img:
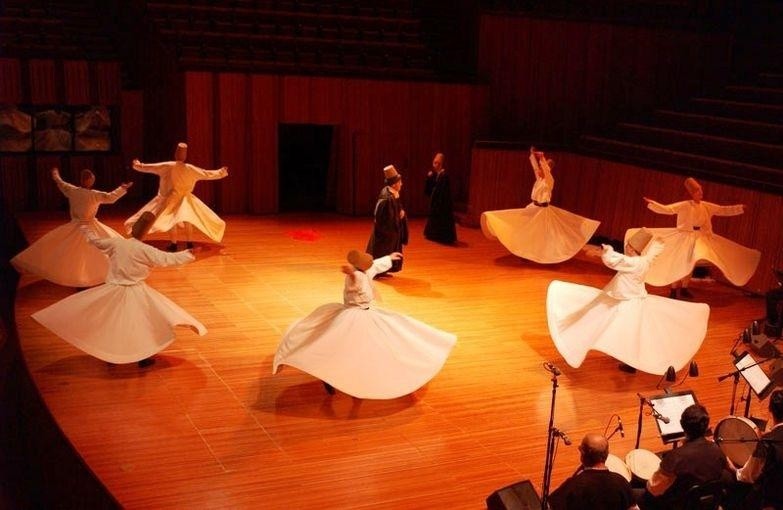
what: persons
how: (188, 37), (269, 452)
(425, 153), (456, 244)
(271, 251), (456, 399)
(124, 143), (229, 251)
(29, 211), (207, 368)
(367, 164), (409, 280)
(622, 177), (762, 300)
(632, 403), (726, 509)
(545, 431), (632, 509)
(547, 227), (712, 375)
(727, 387), (783, 510)
(480, 145), (601, 265)
(10, 167), (134, 292)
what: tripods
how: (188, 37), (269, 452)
(741, 388), (752, 418)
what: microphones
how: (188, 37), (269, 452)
(645, 409), (668, 424)
(543, 362), (561, 376)
(551, 428), (573, 446)
(617, 416), (624, 437)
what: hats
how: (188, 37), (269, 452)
(174, 142), (188, 160)
(132, 211), (156, 241)
(80, 169), (95, 188)
(628, 227), (653, 253)
(383, 165), (399, 179)
(539, 159), (555, 172)
(684, 177), (701, 195)
(347, 250), (374, 272)
